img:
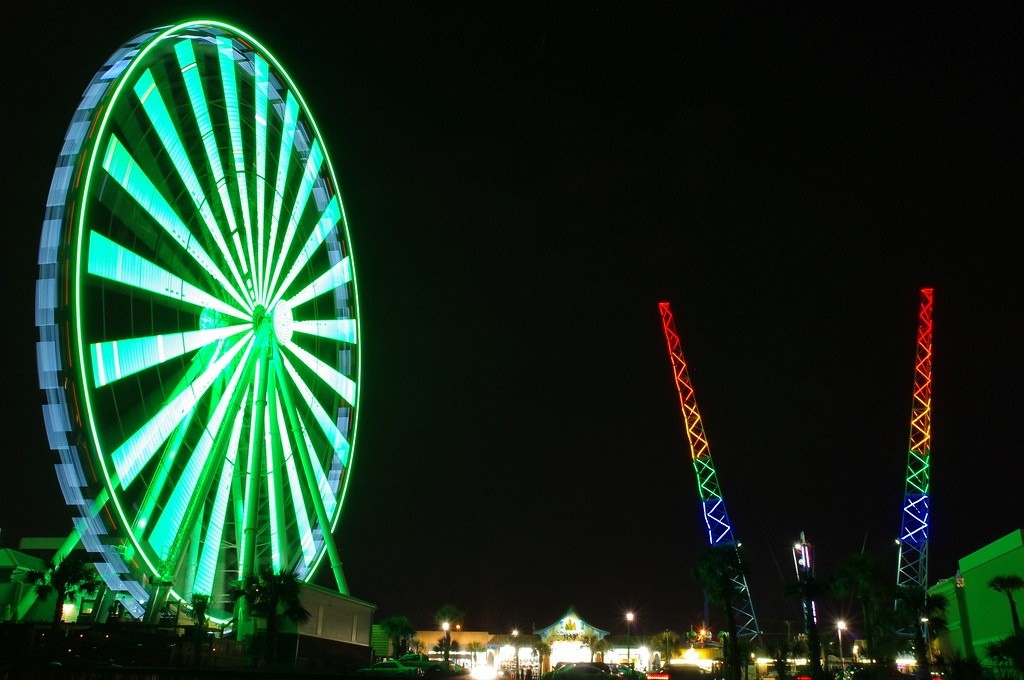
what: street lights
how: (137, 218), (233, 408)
(442, 622), (450, 662)
(511, 628), (520, 680)
(836, 618), (846, 668)
(626, 611), (633, 662)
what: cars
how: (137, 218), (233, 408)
(359, 654), (463, 680)
(542, 661), (715, 680)
(835, 662), (912, 680)
